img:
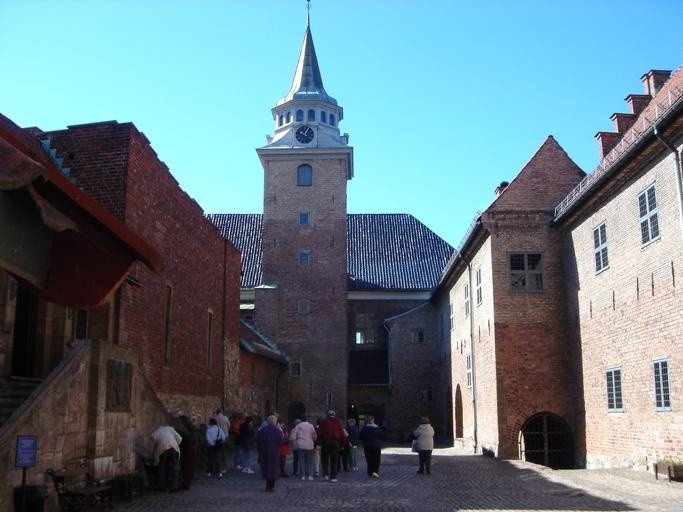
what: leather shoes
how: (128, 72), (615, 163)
(265, 487), (274, 492)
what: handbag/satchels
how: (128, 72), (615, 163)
(216, 439), (223, 450)
(412, 439), (416, 452)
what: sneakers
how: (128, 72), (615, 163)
(301, 476), (305, 480)
(368, 472), (379, 478)
(237, 464), (255, 474)
(324, 474), (338, 482)
(417, 470), (430, 474)
(309, 476), (313, 480)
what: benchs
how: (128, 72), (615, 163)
(47, 466), (114, 511)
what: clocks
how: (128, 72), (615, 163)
(289, 123), (319, 150)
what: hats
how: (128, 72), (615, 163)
(327, 410), (335, 415)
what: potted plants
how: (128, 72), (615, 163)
(653, 458), (683, 481)
(117, 471), (145, 501)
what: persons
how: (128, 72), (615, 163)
(150, 409), (434, 492)
